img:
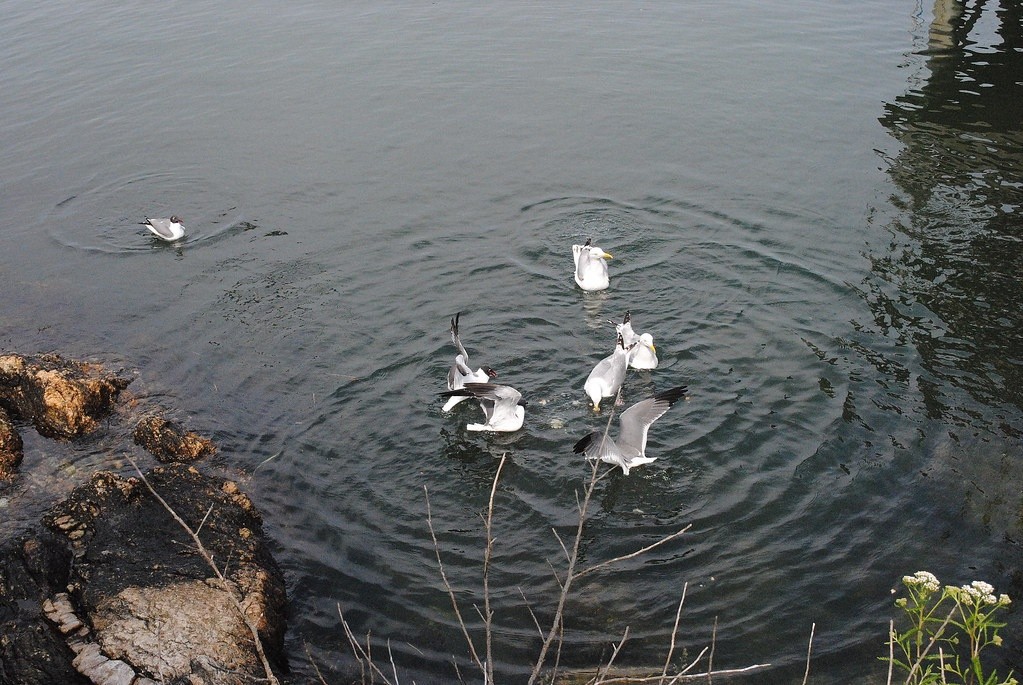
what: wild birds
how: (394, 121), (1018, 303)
(435, 382), (528, 437)
(583, 332), (638, 411)
(572, 238), (614, 292)
(436, 311), (496, 412)
(607, 310), (658, 369)
(138, 215), (186, 242)
(573, 385), (689, 476)
